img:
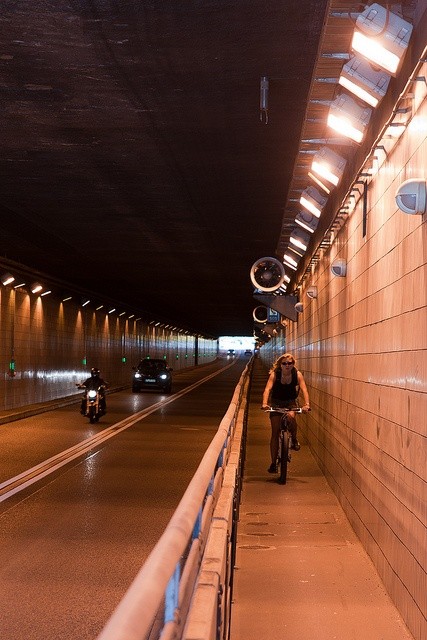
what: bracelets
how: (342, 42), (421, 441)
(304, 403), (310, 406)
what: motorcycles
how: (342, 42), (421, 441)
(76, 384), (105, 423)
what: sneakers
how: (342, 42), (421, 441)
(292, 439), (300, 451)
(268, 463), (277, 473)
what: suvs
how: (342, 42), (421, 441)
(132, 359), (172, 392)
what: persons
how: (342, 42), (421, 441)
(80, 367), (108, 415)
(261, 353), (311, 473)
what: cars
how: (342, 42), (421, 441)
(244, 349), (252, 356)
(227, 349), (235, 355)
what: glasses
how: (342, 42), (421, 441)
(281, 361), (293, 365)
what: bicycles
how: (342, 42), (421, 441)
(260, 407), (311, 483)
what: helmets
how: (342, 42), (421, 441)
(91, 368), (101, 377)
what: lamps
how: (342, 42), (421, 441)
(290, 228), (310, 252)
(350, 4), (413, 79)
(295, 211), (319, 234)
(328, 94), (372, 145)
(339, 56), (391, 109)
(311, 147), (347, 188)
(300, 186), (329, 218)
(283, 250), (301, 268)
